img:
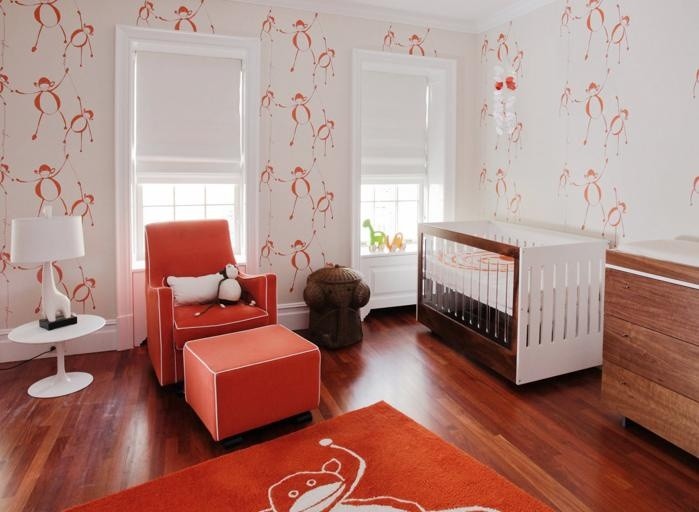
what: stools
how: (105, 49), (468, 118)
(182, 323), (321, 450)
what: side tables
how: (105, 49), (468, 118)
(7, 313), (106, 399)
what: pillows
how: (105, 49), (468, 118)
(163, 272), (224, 305)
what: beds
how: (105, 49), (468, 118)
(415, 219), (610, 387)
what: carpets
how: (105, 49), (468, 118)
(60, 399), (559, 512)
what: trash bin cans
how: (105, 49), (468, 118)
(303, 264), (369, 350)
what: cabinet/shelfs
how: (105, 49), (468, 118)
(600, 235), (698, 458)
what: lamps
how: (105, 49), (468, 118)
(8, 204), (85, 330)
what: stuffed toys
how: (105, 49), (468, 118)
(195, 263), (256, 317)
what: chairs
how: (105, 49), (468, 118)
(144, 219), (277, 387)
